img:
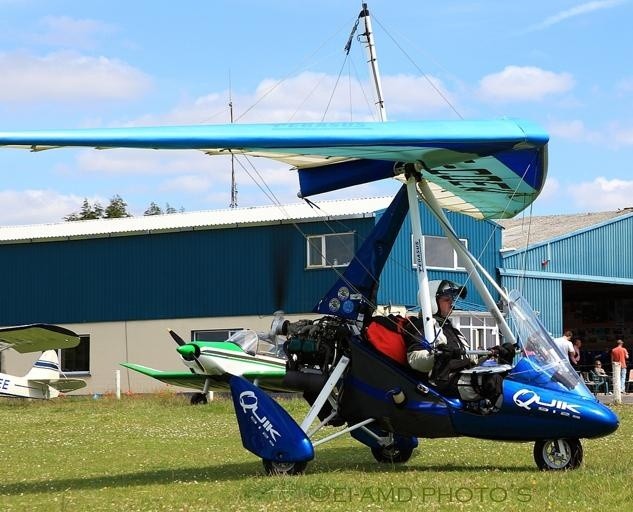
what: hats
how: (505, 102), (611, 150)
(617, 340), (624, 344)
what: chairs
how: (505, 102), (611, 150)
(576, 368), (633, 398)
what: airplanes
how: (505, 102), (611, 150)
(115, 324), (306, 405)
(0, 323), (89, 404)
(0, 3), (620, 477)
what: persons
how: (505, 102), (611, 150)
(609, 339), (629, 396)
(404, 277), (514, 418)
(593, 359), (608, 378)
(569, 338), (582, 371)
(592, 344), (612, 363)
(553, 329), (577, 366)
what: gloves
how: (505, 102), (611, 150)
(491, 345), (507, 359)
(446, 347), (465, 361)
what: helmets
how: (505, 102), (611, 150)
(417, 279), (467, 315)
